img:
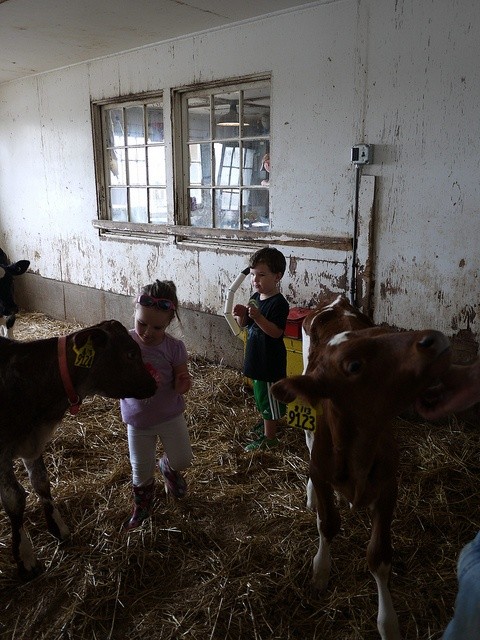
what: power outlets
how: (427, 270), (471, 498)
(351, 144), (373, 164)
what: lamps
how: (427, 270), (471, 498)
(216, 102), (248, 127)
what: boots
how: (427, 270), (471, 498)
(129, 478), (155, 528)
(159, 450), (187, 498)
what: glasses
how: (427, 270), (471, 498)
(140, 295), (176, 312)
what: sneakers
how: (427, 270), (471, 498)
(254, 424), (283, 437)
(243, 435), (278, 453)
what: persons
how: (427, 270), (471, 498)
(119, 280), (192, 531)
(233, 248), (289, 453)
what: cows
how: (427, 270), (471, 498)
(267, 293), (454, 640)
(0, 318), (158, 578)
(0, 247), (31, 340)
